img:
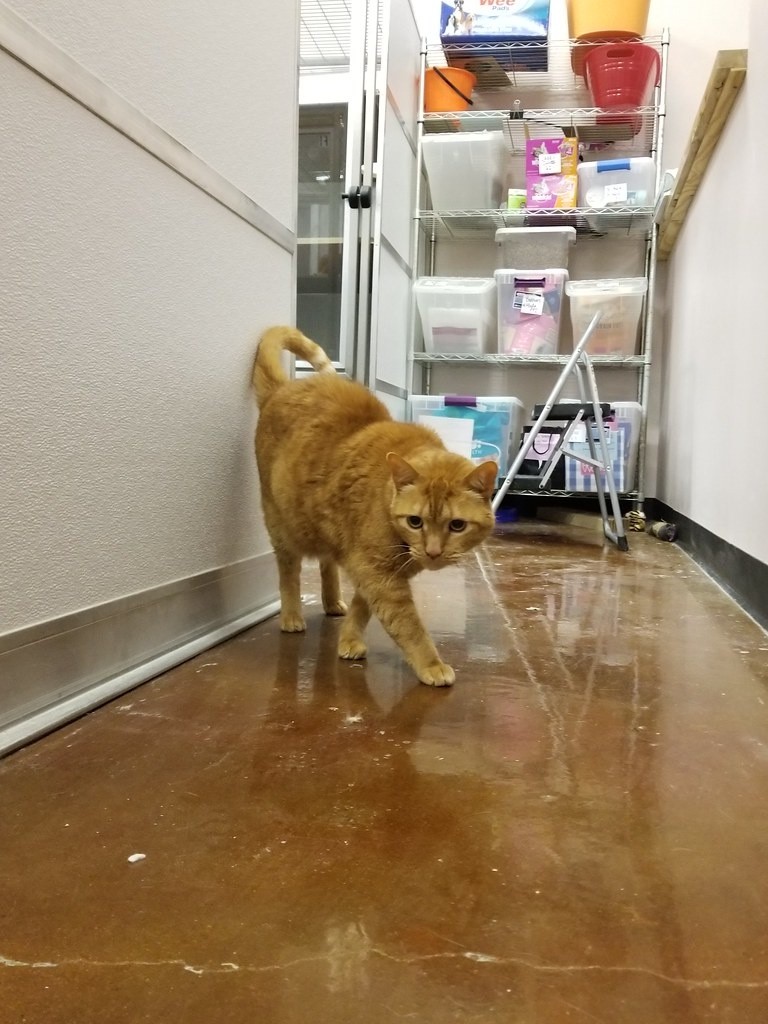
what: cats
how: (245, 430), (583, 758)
(252, 327), (497, 688)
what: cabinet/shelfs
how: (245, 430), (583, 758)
(405, 27), (669, 534)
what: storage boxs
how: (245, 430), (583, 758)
(492, 267), (570, 355)
(439, 0), (550, 72)
(526, 177), (578, 209)
(494, 226), (576, 272)
(418, 131), (509, 236)
(407, 394), (524, 476)
(566, 0), (650, 76)
(576, 155), (657, 226)
(563, 277), (649, 355)
(582, 43), (660, 124)
(559, 398), (642, 492)
(525, 137), (578, 176)
(411, 275), (497, 353)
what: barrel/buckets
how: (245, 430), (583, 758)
(565, 0), (650, 76)
(424, 66), (476, 112)
(581, 44), (661, 134)
